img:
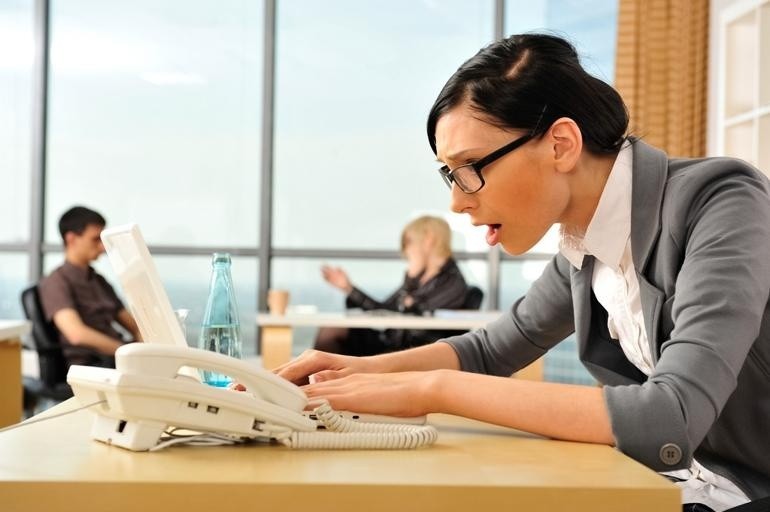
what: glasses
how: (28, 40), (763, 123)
(438, 129), (541, 194)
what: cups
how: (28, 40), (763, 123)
(265, 289), (289, 318)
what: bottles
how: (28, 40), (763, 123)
(198, 253), (241, 388)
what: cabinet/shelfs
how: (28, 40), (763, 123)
(0, 394), (683, 511)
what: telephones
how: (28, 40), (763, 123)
(67, 342), (316, 451)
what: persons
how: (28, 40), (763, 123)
(310, 214), (469, 357)
(37, 205), (145, 373)
(260, 32), (769, 512)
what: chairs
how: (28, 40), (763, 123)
(22, 286), (75, 400)
(412, 287), (482, 347)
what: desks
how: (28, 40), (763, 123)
(256, 311), (543, 380)
(1, 320), (32, 428)
